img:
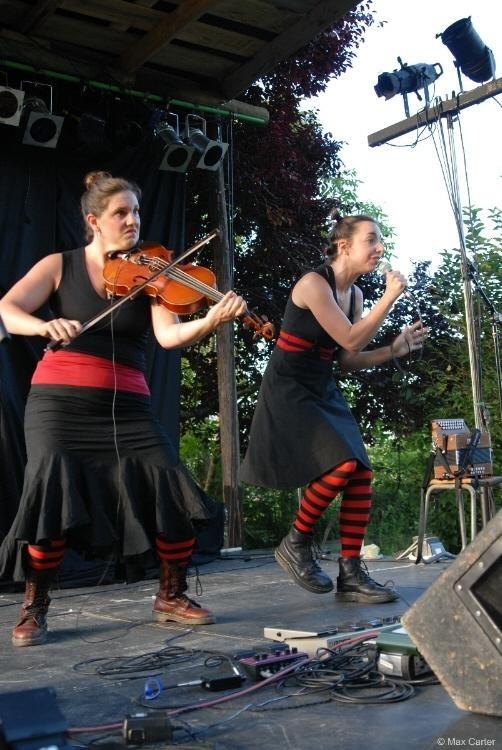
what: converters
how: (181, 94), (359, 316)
(201, 673), (242, 692)
(124, 712), (172, 745)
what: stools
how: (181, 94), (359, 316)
(414, 474), (502, 565)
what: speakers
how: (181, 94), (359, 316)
(0, 688), (69, 750)
(401, 508), (502, 716)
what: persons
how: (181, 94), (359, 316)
(237, 211), (435, 607)
(0, 170), (249, 647)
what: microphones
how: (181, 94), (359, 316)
(379, 262), (416, 303)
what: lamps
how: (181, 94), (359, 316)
(368, 16), (502, 147)
(0, 58), (266, 173)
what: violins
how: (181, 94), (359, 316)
(104, 241), (277, 338)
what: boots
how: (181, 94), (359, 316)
(10, 567), (53, 647)
(150, 558), (219, 626)
(273, 523), (334, 594)
(334, 556), (402, 604)
